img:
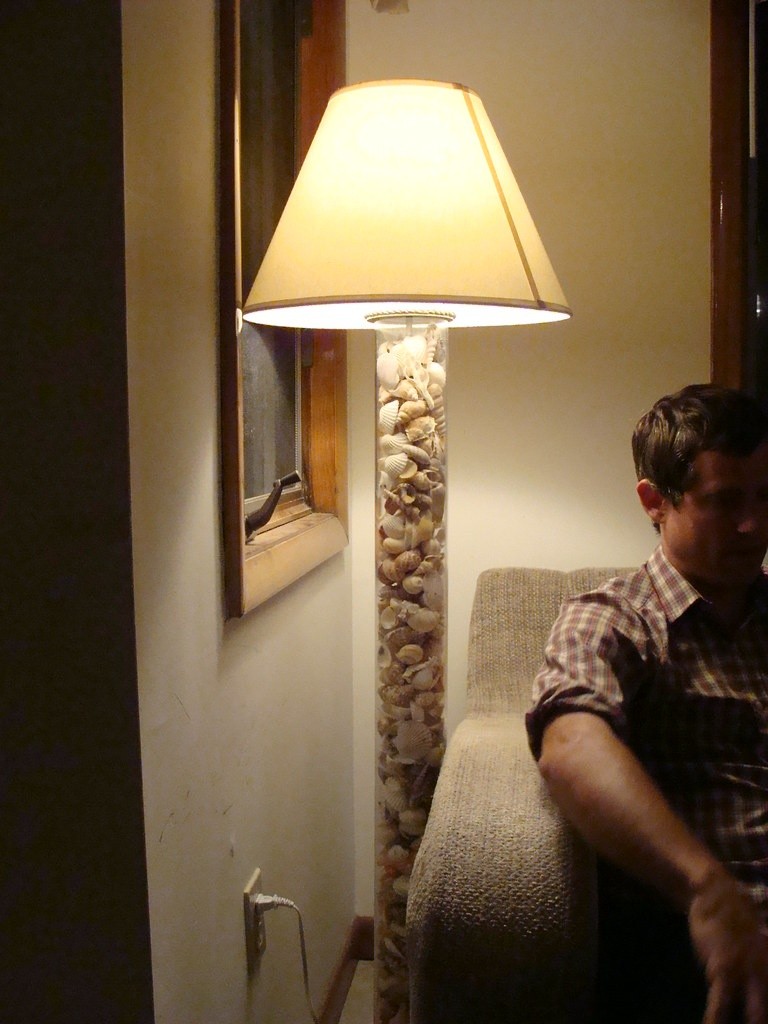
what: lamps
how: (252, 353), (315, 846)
(245, 80), (566, 1015)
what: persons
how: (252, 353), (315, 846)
(526, 383), (768, 1024)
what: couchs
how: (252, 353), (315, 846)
(406, 568), (654, 1021)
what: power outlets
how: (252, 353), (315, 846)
(243, 867), (275, 970)
